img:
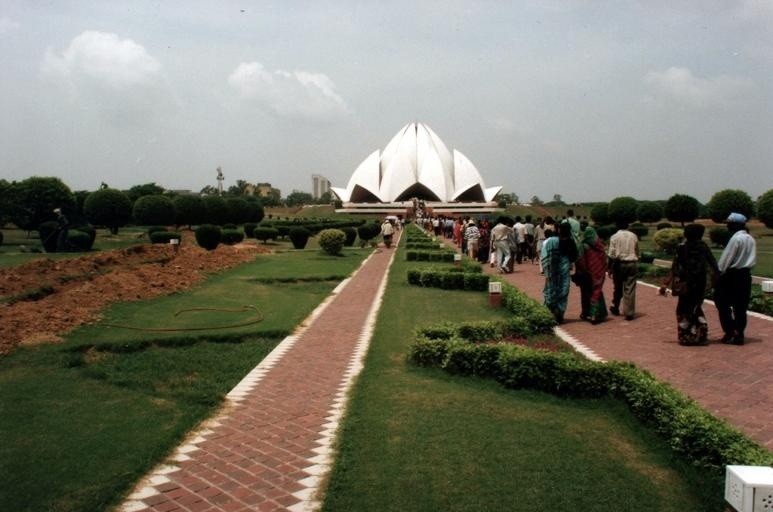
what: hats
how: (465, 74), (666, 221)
(726, 213), (746, 223)
(468, 219), (475, 225)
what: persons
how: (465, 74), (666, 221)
(540, 226), (578, 323)
(608, 216), (643, 321)
(715, 211), (757, 346)
(379, 195), (600, 275)
(570, 225), (608, 325)
(654, 222), (720, 346)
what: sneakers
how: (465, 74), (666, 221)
(610, 306), (619, 315)
(720, 331), (744, 345)
(591, 318), (597, 325)
(625, 315), (633, 320)
